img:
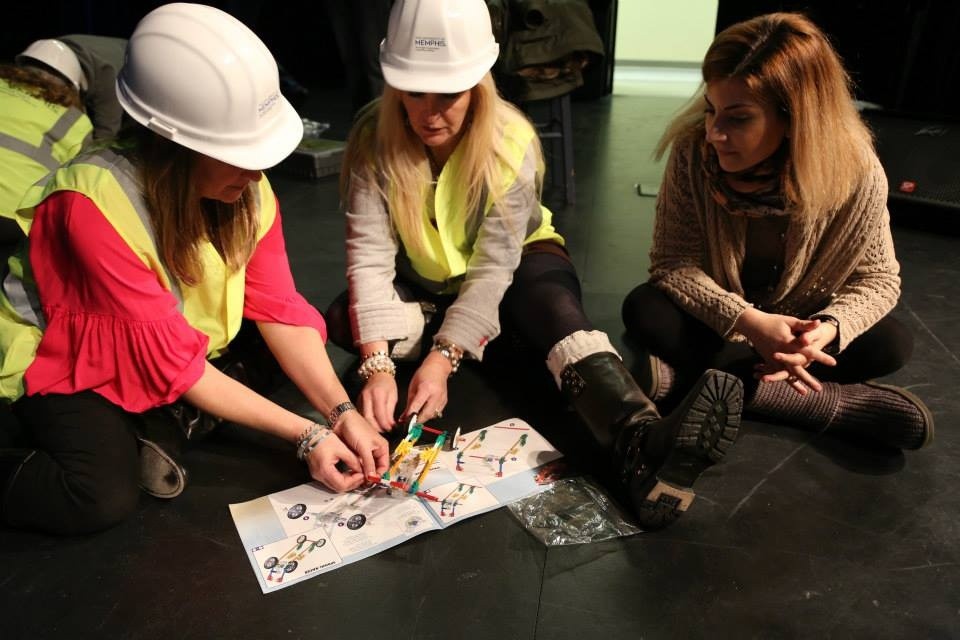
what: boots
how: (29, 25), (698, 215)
(138, 344), (253, 498)
(743, 374), (933, 451)
(390, 300), (532, 364)
(645, 354), (696, 405)
(543, 327), (743, 529)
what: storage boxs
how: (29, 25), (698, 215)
(884, 134), (959, 242)
(268, 135), (347, 180)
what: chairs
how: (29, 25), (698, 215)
(486, 1), (585, 208)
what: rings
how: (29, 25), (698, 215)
(435, 408), (443, 419)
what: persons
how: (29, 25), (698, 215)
(0, 37), (96, 292)
(52, 32), (131, 153)
(0, 6), (389, 539)
(326, 0), (746, 535)
(620, 16), (935, 453)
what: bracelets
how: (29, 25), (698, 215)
(296, 422), (333, 470)
(324, 402), (358, 428)
(358, 351), (397, 382)
(427, 336), (464, 381)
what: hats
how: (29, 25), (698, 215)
(15, 38), (81, 97)
(380, 0), (501, 94)
(116, 2), (304, 169)
